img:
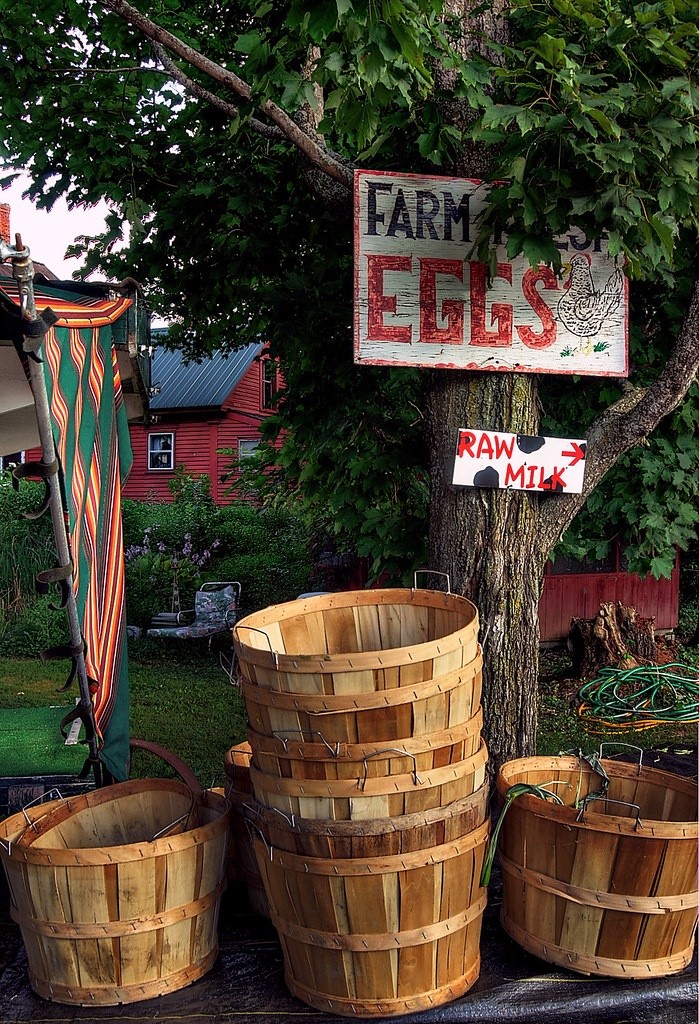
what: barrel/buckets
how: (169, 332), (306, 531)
(241, 772), (487, 860)
(231, 589), (480, 694)
(244, 703), (482, 780)
(249, 736), (489, 821)
(16, 778), (197, 848)
(0, 778), (232, 1008)
(247, 818), (490, 1017)
(235, 644), (484, 743)
(495, 758), (698, 979)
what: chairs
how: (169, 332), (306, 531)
(147, 581), (256, 655)
